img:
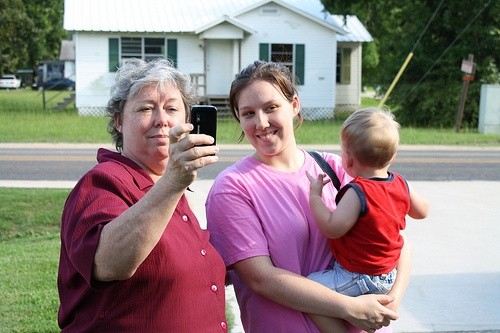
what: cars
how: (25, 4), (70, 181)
(0, 75), (21, 90)
(31, 78), (76, 91)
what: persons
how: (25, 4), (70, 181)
(303, 107), (429, 333)
(204, 59), (411, 333)
(57, 57), (228, 333)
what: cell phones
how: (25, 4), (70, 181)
(189, 105), (217, 157)
